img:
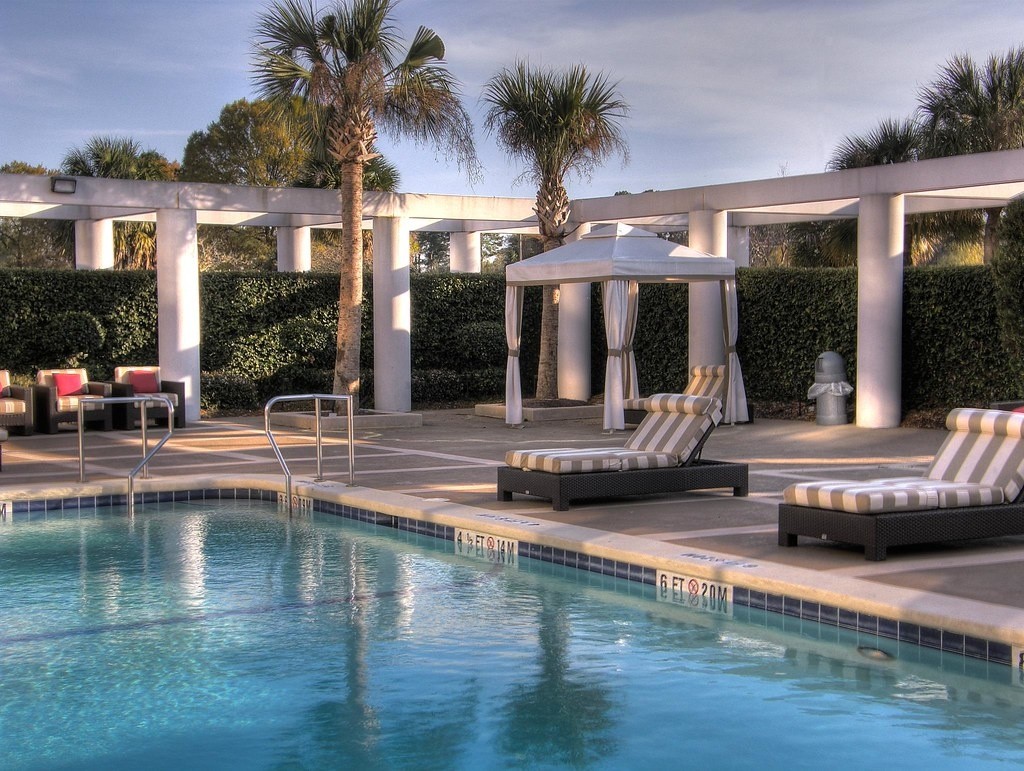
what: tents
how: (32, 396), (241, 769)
(505, 221), (750, 427)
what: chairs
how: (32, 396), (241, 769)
(0, 370), (33, 437)
(35, 367), (113, 434)
(497, 393), (749, 511)
(624, 364), (726, 428)
(777, 408), (1024, 562)
(104, 366), (186, 431)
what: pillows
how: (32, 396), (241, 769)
(52, 373), (82, 396)
(0, 381), (4, 399)
(128, 371), (159, 393)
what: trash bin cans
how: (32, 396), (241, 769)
(813, 351), (849, 424)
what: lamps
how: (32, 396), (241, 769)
(50, 176), (77, 194)
